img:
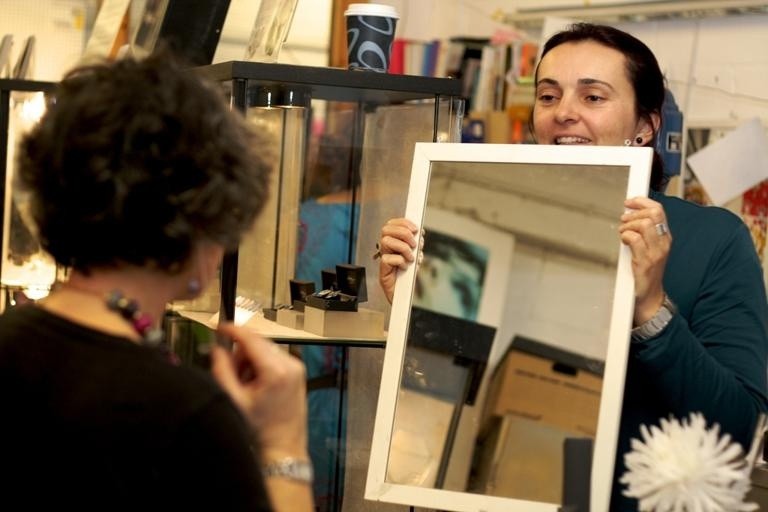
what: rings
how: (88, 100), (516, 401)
(369, 241), (384, 258)
(652, 222), (670, 238)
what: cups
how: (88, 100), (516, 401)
(343, 3), (399, 76)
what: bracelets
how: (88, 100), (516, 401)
(630, 292), (681, 345)
(244, 452), (320, 485)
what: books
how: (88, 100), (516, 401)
(386, 31), (541, 118)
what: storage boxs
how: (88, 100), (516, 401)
(481, 334), (606, 440)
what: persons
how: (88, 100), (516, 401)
(1, 55), (317, 511)
(377, 21), (768, 510)
(287, 134), (363, 512)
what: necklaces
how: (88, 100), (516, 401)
(41, 275), (184, 372)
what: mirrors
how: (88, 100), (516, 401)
(362, 139), (656, 512)
